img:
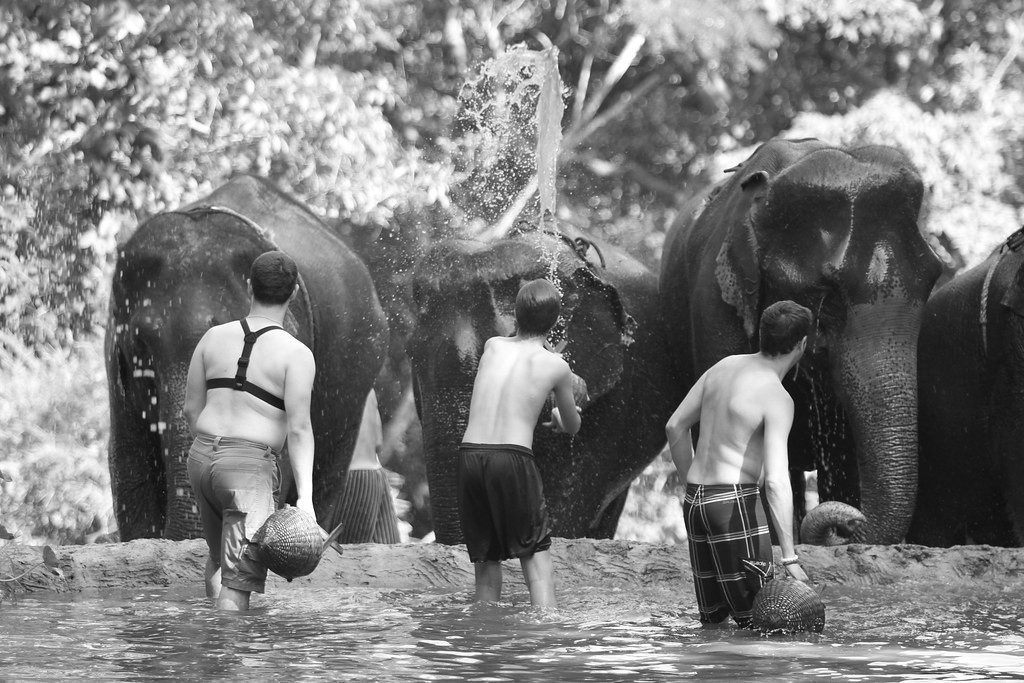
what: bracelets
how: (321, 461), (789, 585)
(781, 556), (798, 566)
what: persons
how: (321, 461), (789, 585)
(182, 251), (318, 611)
(456, 279), (582, 613)
(329, 386), (400, 544)
(666, 300), (814, 628)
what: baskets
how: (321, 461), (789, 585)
(751, 577), (825, 632)
(257, 505), (322, 580)
(544, 372), (589, 417)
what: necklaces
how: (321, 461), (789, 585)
(248, 315), (283, 327)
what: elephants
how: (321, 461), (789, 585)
(102, 175), (389, 542)
(405, 209), (691, 544)
(905, 224), (1024, 547)
(661, 137), (944, 546)
